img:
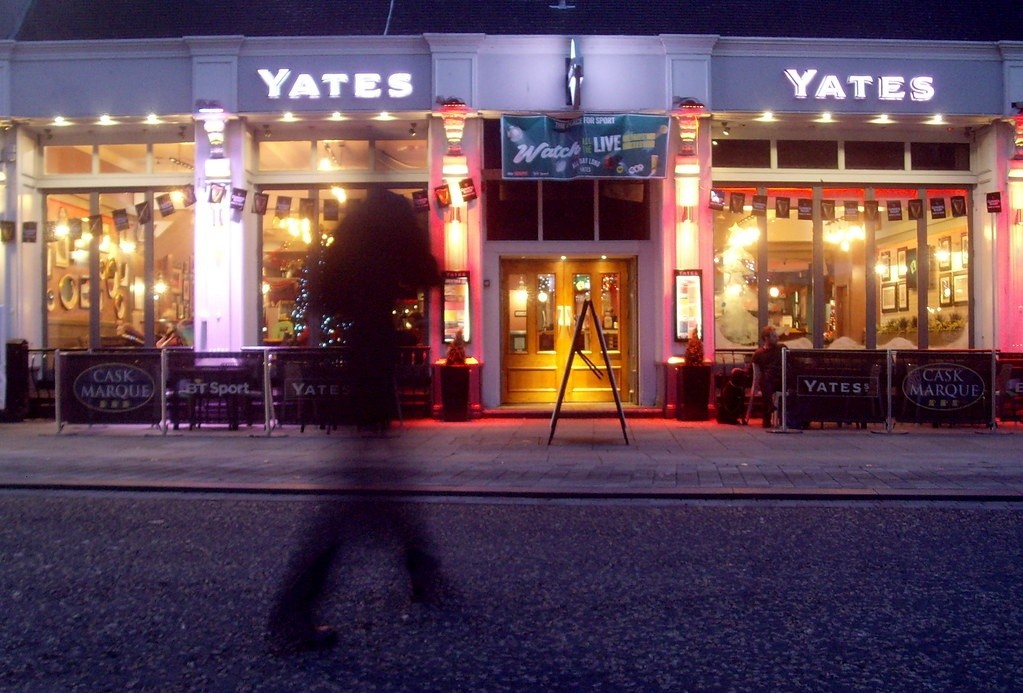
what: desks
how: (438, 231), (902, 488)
(172, 366), (249, 431)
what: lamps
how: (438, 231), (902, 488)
(409, 122), (417, 136)
(681, 205), (693, 224)
(450, 206), (461, 224)
(1014, 209), (1023, 225)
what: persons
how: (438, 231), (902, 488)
(270, 184), (466, 652)
(752, 324), (788, 429)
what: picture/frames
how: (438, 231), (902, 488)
(938, 272), (954, 308)
(881, 250), (891, 282)
(960, 232), (968, 268)
(54, 238), (71, 269)
(59, 274), (80, 311)
(937, 235), (952, 271)
(100, 223), (137, 320)
(47, 244), (53, 281)
(171, 255), (194, 320)
(78, 275), (90, 309)
(881, 282), (898, 314)
(897, 280), (909, 312)
(897, 246), (908, 279)
(952, 270), (968, 307)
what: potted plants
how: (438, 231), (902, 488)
(432, 326), (479, 422)
(674, 326), (716, 422)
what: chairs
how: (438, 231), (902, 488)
(745, 362), (789, 429)
